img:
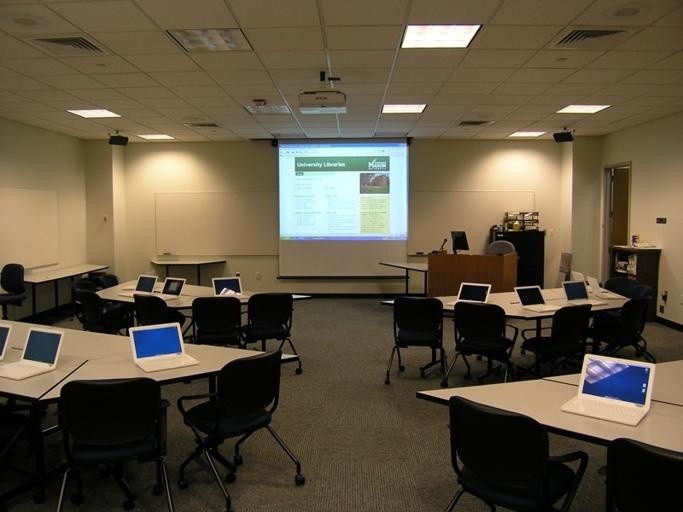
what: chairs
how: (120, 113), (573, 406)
(50, 373), (180, 512)
(384, 277), (657, 388)
(174, 348), (306, 511)
(443, 395), (590, 512)
(2, 260), (308, 377)
(605, 437), (683, 512)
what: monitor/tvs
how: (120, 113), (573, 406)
(451, 231), (469, 254)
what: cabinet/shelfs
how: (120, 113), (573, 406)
(489, 226), (546, 290)
(610, 244), (662, 323)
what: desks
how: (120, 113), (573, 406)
(415, 358), (683, 478)
(1, 309), (301, 493)
(150, 255), (227, 286)
(378, 260), (430, 297)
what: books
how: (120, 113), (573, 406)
(626, 254), (637, 281)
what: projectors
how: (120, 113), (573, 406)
(298, 88), (347, 114)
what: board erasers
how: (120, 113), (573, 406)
(163, 253), (172, 254)
(416, 252), (425, 255)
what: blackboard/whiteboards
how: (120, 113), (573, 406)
(0, 188), (60, 275)
(155, 192), (280, 255)
(407, 191), (536, 255)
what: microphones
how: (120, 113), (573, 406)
(440, 238), (447, 250)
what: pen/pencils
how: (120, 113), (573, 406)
(11, 346), (24, 352)
(511, 302), (519, 304)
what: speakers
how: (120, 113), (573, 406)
(554, 132), (573, 143)
(109, 135), (128, 146)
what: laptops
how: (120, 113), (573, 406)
(0, 328), (63, 381)
(562, 281), (608, 307)
(119, 274), (157, 298)
(129, 322), (199, 373)
(587, 275), (626, 299)
(514, 285), (562, 312)
(212, 277), (243, 298)
(0, 325), (11, 361)
(153, 277), (186, 301)
(447, 282), (491, 306)
(571, 270), (608, 293)
(561, 354), (655, 427)
